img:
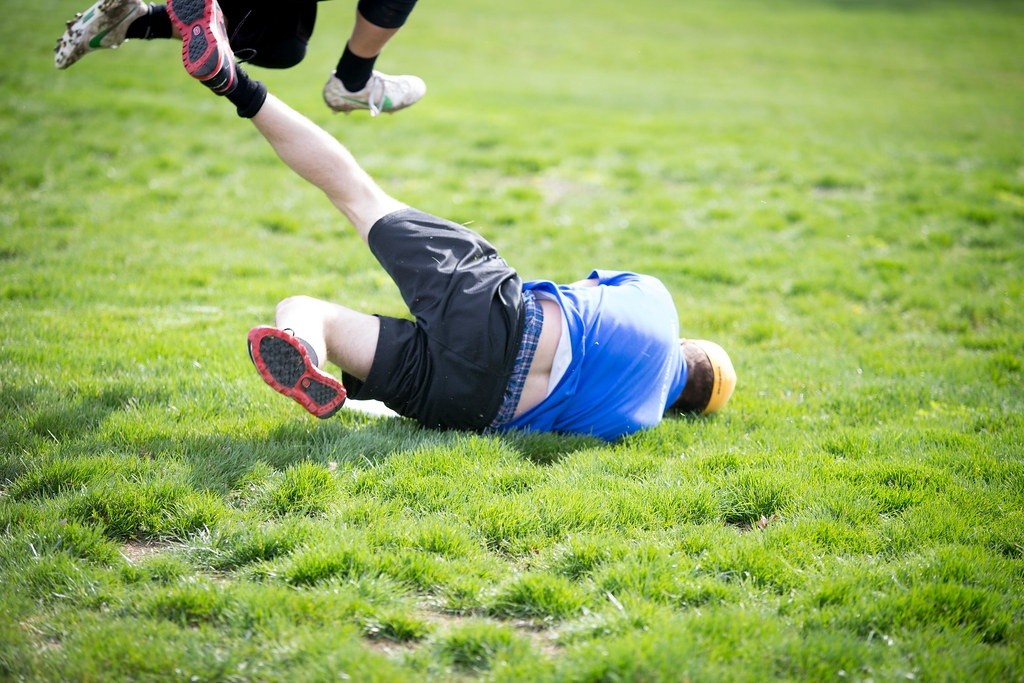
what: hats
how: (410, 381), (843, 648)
(696, 338), (737, 416)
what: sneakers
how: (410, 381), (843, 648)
(323, 70), (427, 115)
(164, 0), (238, 96)
(247, 325), (346, 419)
(52, 0), (149, 71)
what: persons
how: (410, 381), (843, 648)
(166, 0), (739, 443)
(53, 0), (427, 117)
